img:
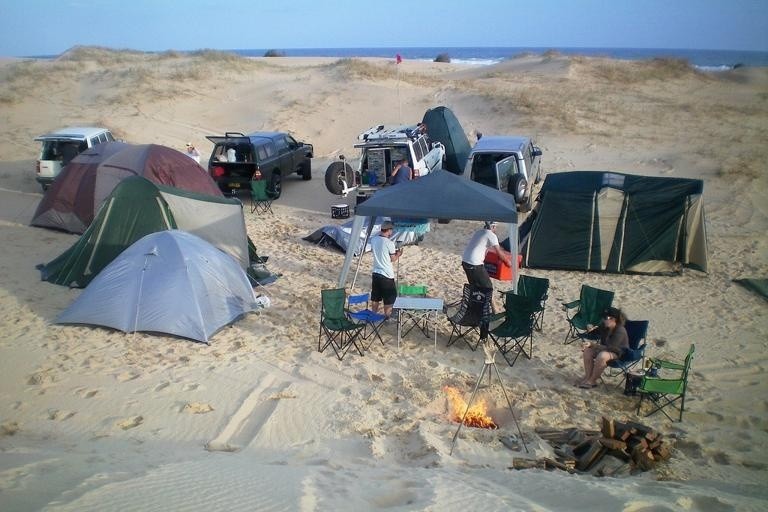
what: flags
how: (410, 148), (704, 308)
(396, 54), (402, 64)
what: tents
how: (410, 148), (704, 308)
(25, 139), (227, 238)
(30, 174), (271, 292)
(497, 169), (709, 278)
(45, 228), (262, 349)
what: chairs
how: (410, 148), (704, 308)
(249, 179), (279, 214)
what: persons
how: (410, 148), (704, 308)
(59, 142), (81, 168)
(459, 221), (513, 314)
(572, 307), (630, 389)
(389, 158), (413, 185)
(367, 220), (404, 325)
(183, 141), (201, 164)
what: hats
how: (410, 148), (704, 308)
(381, 221), (395, 230)
(185, 142), (192, 147)
(598, 308), (621, 318)
(485, 221), (499, 227)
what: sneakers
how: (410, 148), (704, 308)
(573, 381), (597, 389)
(385, 317), (397, 325)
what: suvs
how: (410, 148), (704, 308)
(33, 125), (123, 190)
(205, 129), (314, 203)
(461, 132), (545, 214)
(323, 121), (446, 196)
